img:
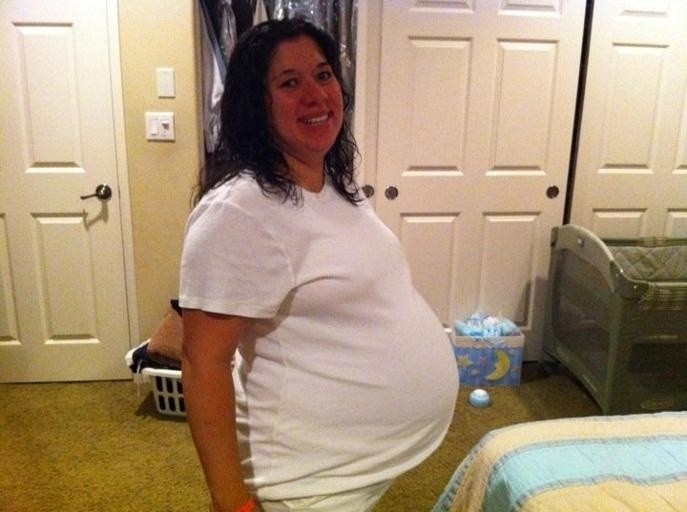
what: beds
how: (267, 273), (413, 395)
(540, 222), (685, 414)
(426, 411), (687, 511)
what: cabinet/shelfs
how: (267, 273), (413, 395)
(564, 0), (686, 236)
(197, 0), (590, 366)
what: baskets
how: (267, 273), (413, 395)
(125, 338), (188, 418)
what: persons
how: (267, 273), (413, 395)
(177, 16), (461, 512)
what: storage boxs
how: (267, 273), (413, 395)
(447, 312), (525, 386)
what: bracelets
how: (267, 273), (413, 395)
(235, 497), (255, 511)
